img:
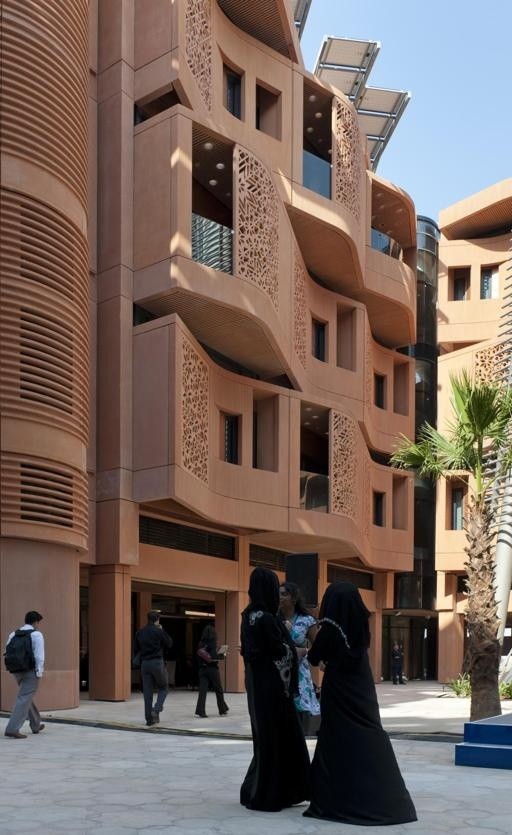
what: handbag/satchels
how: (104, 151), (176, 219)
(197, 647), (212, 664)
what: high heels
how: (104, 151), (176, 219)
(200, 714), (209, 718)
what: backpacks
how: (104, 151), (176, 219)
(2, 628), (40, 674)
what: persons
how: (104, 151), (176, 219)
(136, 611), (173, 726)
(278, 579), (321, 719)
(301, 575), (418, 826)
(391, 641), (407, 685)
(239, 567), (309, 812)
(195, 622), (230, 718)
(3, 611), (46, 739)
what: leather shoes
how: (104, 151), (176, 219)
(33, 724), (45, 735)
(147, 710), (159, 725)
(4, 731), (27, 739)
